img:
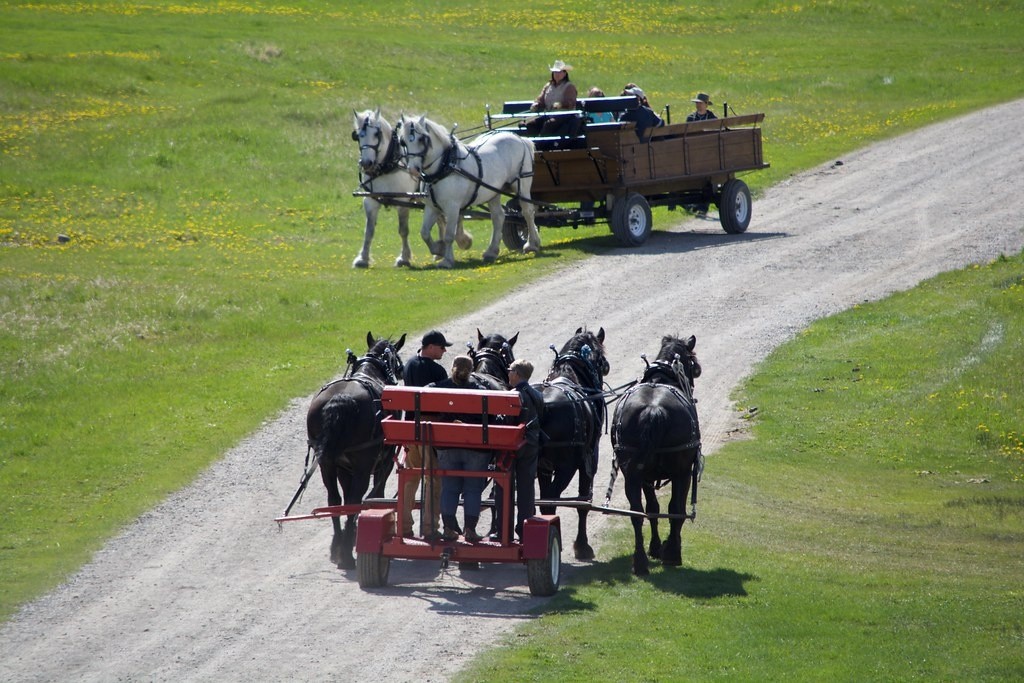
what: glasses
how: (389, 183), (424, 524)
(429, 343), (445, 350)
(507, 368), (516, 374)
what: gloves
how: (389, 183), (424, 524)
(530, 103), (542, 113)
(552, 102), (562, 109)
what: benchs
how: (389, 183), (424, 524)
(381, 386), (528, 450)
(503, 95), (639, 131)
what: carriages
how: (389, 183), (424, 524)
(352, 96), (770, 269)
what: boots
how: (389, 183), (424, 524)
(464, 514), (483, 540)
(442, 519), (458, 540)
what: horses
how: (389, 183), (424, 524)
(472, 322), (611, 559)
(350, 103), (542, 269)
(610, 332), (702, 576)
(305, 330), (408, 571)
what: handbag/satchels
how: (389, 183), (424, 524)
(538, 428), (550, 446)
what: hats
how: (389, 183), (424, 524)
(624, 87), (644, 100)
(453, 356), (473, 373)
(422, 330), (453, 347)
(550, 60), (574, 72)
(692, 93), (713, 105)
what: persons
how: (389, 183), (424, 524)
(397, 330), (544, 545)
(686, 93), (720, 214)
(518, 61), (665, 150)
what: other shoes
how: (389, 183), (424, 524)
(489, 532), (514, 542)
(425, 530), (441, 542)
(404, 531), (414, 539)
